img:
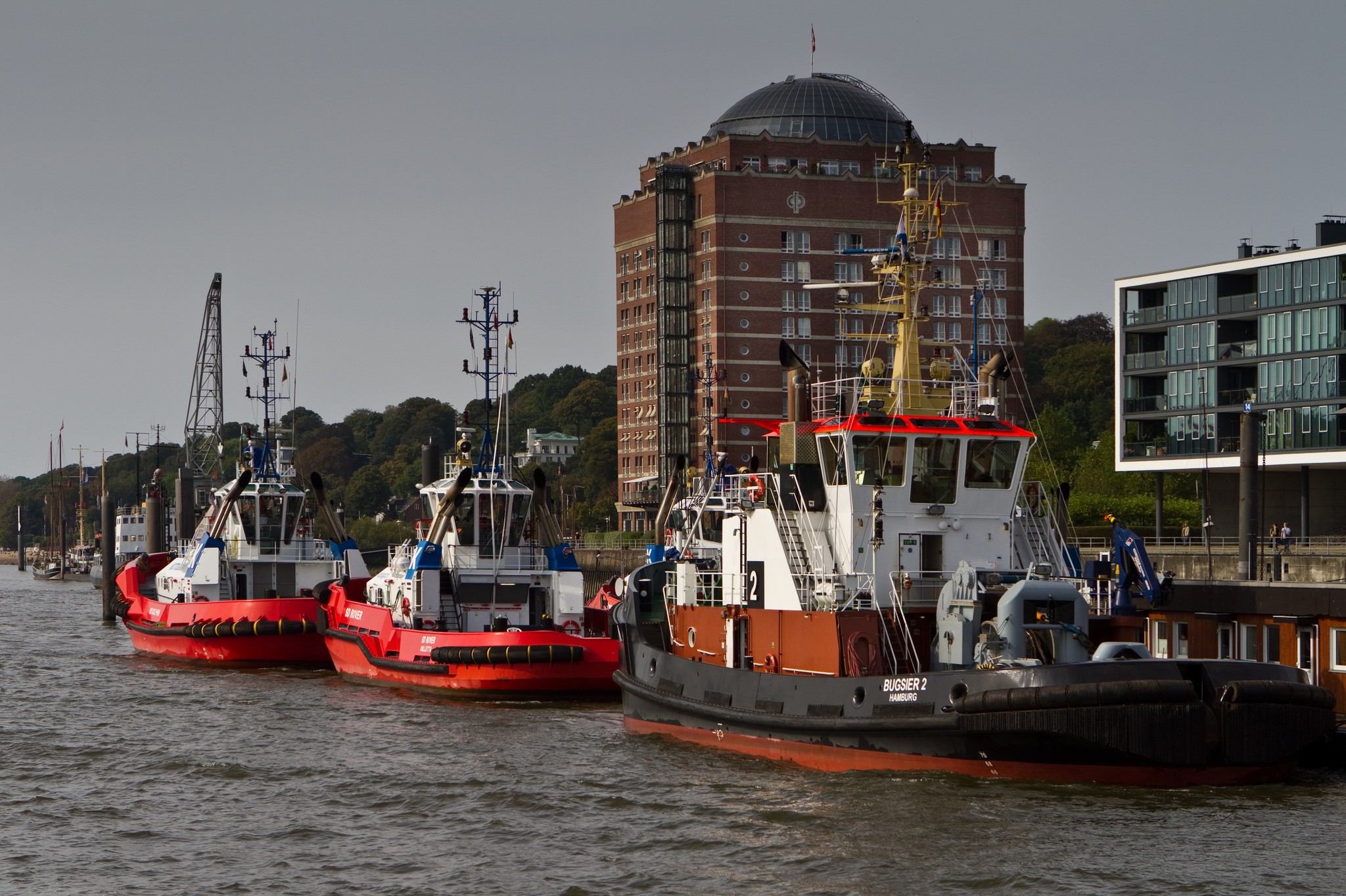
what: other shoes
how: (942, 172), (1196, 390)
(1287, 548), (1289, 552)
(1276, 548), (1279, 551)
(1284, 551), (1286, 552)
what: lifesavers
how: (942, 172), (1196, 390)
(690, 554), (693, 559)
(415, 519), (433, 538)
(295, 517), (310, 534)
(562, 620), (580, 635)
(194, 595), (210, 603)
(522, 520), (535, 540)
(665, 528), (672, 544)
(421, 620), (436, 632)
(747, 474), (763, 504)
(683, 549), (691, 561)
(763, 653), (779, 674)
(208, 516), (216, 530)
(402, 597), (410, 618)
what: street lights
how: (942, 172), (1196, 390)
(605, 518), (609, 532)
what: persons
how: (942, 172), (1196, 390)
(1269, 522), (1292, 552)
(1182, 523), (1190, 546)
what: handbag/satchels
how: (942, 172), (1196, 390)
(1286, 534), (1290, 540)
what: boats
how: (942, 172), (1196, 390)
(90, 273), (226, 595)
(32, 420), (115, 582)
(313, 281), (626, 699)
(110, 317), (372, 667)
(612, 108), (1346, 788)
